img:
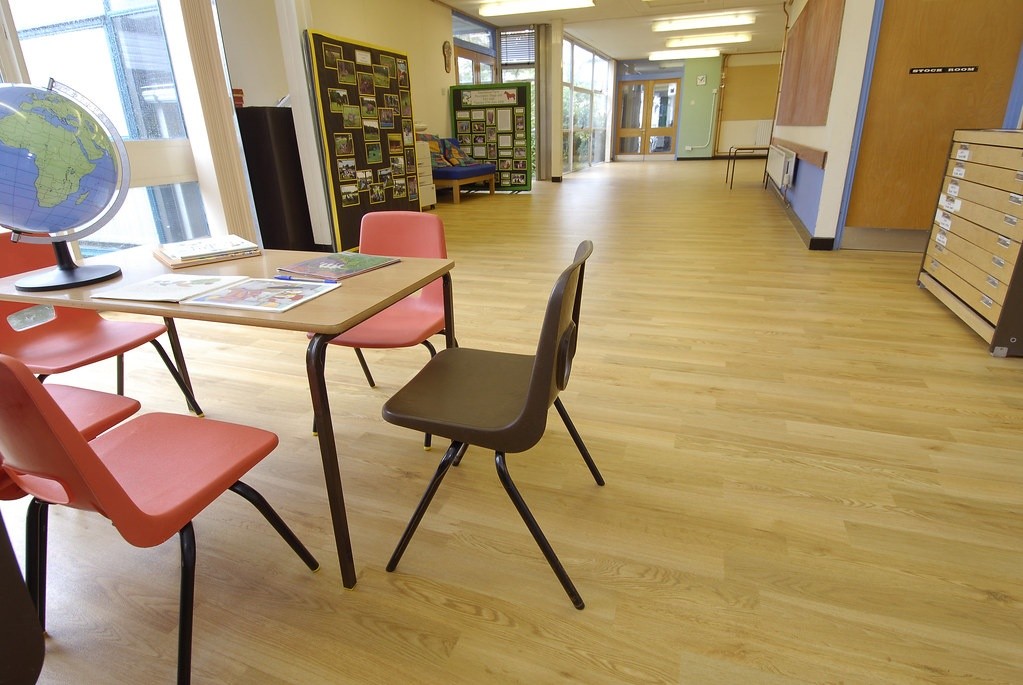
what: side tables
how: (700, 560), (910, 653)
(414, 141), (437, 209)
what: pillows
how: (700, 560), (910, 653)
(443, 139), (480, 168)
(415, 132), (453, 168)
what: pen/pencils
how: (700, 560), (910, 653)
(275, 274), (338, 285)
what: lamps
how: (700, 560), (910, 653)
(647, 47), (723, 61)
(651, 9), (761, 32)
(664, 30), (753, 47)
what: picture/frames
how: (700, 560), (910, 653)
(307, 26), (422, 253)
(449, 82), (532, 191)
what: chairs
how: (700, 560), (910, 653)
(0, 229), (206, 418)
(0, 353), (321, 685)
(305, 211), (462, 451)
(385, 238), (606, 612)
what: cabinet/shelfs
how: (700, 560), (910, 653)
(914, 127), (1022, 359)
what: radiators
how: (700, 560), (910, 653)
(765, 143), (797, 191)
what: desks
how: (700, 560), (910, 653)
(725, 146), (770, 191)
(0, 243), (459, 591)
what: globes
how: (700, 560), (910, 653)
(0, 78), (134, 292)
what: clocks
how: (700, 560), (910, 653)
(696, 74), (707, 86)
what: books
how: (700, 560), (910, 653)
(88, 271), (344, 313)
(152, 233), (263, 269)
(276, 250), (400, 281)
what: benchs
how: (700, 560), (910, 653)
(420, 137), (496, 206)
